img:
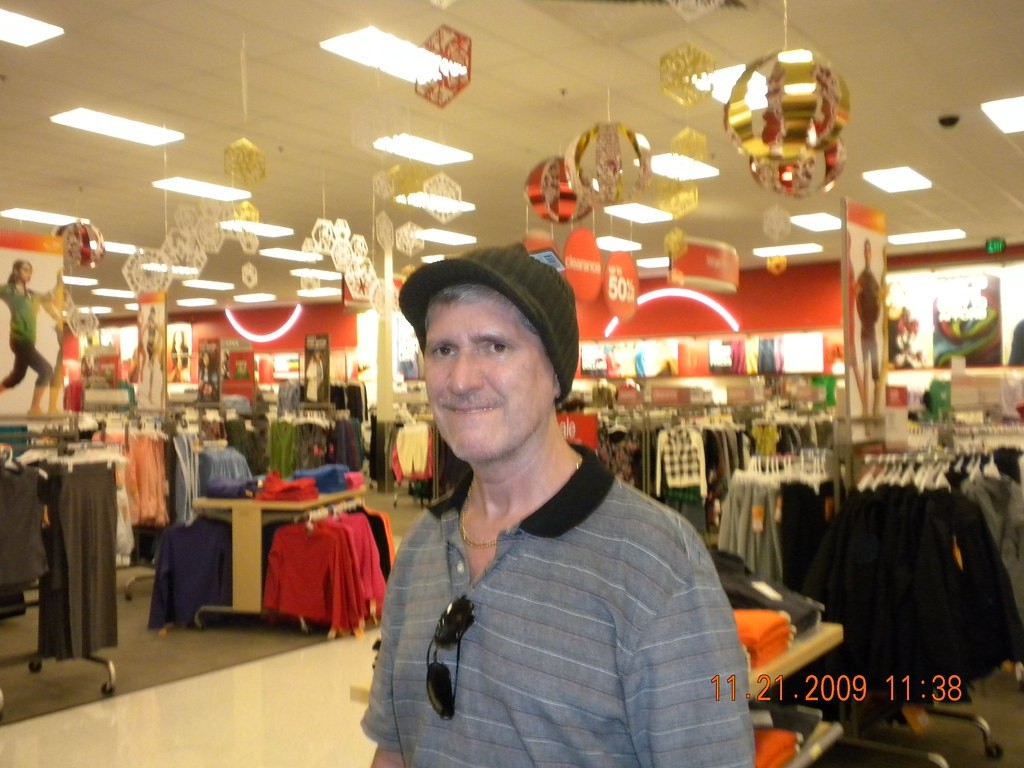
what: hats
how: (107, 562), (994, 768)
(399, 243), (579, 403)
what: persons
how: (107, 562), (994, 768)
(846, 230), (886, 437)
(171, 330), (189, 382)
(0, 259), (64, 418)
(138, 305), (165, 404)
(361, 245), (755, 768)
(221, 351), (230, 380)
(198, 351), (219, 402)
(306, 349), (325, 403)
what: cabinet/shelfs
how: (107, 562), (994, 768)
(747, 620), (844, 768)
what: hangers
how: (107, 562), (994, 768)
(0, 400), (1024, 505)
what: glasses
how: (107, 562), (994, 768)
(426, 595), (476, 721)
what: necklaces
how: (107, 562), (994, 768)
(460, 454), (584, 550)
